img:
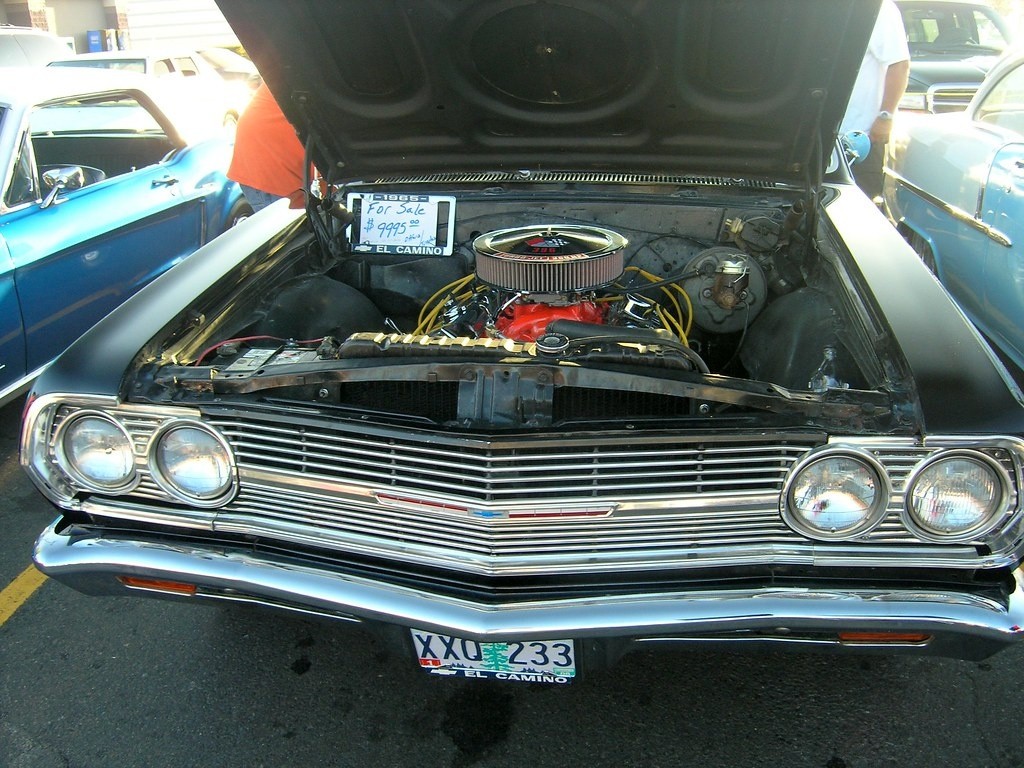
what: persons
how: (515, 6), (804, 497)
(226, 80), (322, 214)
(835, 0), (910, 215)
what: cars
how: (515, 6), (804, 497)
(18, 1), (1024, 684)
(0, 67), (257, 406)
(882, 47), (1024, 371)
(888, 1), (1016, 115)
(48, 50), (220, 106)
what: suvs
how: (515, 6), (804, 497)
(1, 24), (75, 82)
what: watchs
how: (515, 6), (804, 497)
(878, 111), (894, 121)
